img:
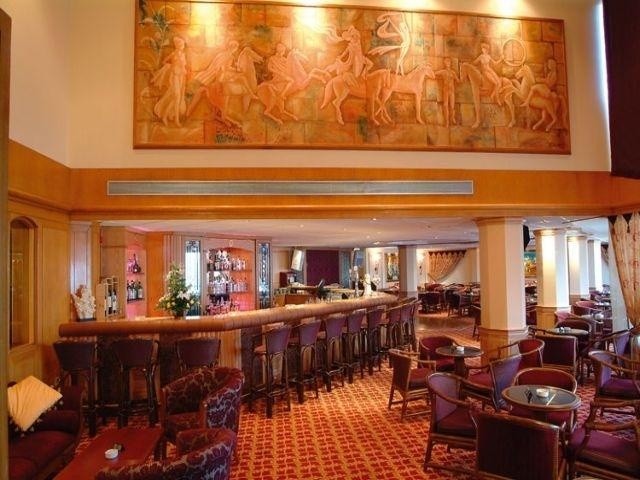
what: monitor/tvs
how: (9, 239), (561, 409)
(319, 279), (325, 288)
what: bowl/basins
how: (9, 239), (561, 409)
(457, 346), (464, 352)
(536, 388), (549, 398)
(104, 449), (118, 459)
(564, 327), (570, 331)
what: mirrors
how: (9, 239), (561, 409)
(184, 239), (200, 316)
(256, 240), (272, 308)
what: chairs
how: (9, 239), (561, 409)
(510, 366), (579, 394)
(384, 345), (437, 422)
(421, 370), (497, 475)
(417, 334), (461, 353)
(472, 409), (568, 479)
(422, 282), (540, 341)
(461, 353), (522, 406)
(523, 282), (639, 356)
(494, 336), (545, 366)
(587, 346), (639, 432)
(569, 397), (639, 480)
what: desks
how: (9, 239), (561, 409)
(500, 380), (583, 422)
(54, 422), (163, 480)
(434, 341), (485, 367)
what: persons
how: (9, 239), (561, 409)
(332, 24), (374, 81)
(194, 39), (260, 101)
(267, 42), (294, 98)
(388, 12), (411, 78)
(472, 42), (506, 107)
(151, 35), (188, 129)
(434, 57), (459, 128)
(518, 58), (558, 108)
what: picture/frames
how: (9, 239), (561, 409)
(524, 250), (536, 278)
(385, 253), (400, 282)
(134, 0), (572, 155)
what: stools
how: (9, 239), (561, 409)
(55, 335), (223, 430)
(237, 282), (421, 420)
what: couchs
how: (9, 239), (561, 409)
(156, 363), (248, 442)
(92, 428), (240, 479)
(6, 380), (84, 480)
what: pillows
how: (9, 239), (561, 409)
(6, 375), (63, 432)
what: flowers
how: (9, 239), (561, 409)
(153, 260), (203, 314)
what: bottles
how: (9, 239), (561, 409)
(126, 252), (145, 302)
(207, 251), (248, 316)
(104, 288), (117, 318)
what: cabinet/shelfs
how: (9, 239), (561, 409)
(101, 225), (148, 321)
(200, 235), (256, 315)
